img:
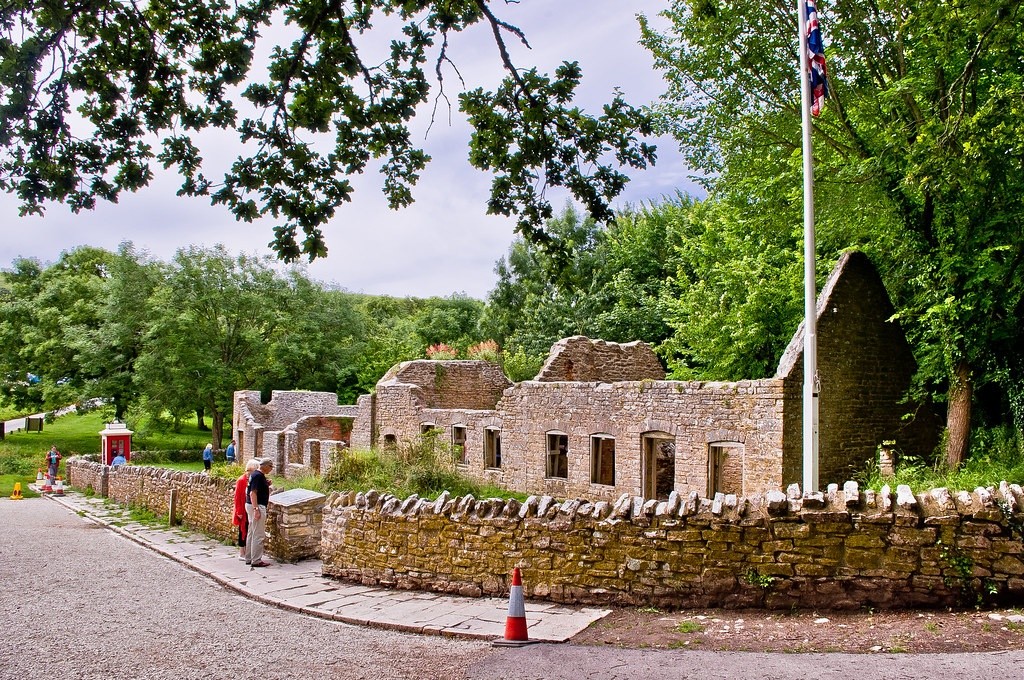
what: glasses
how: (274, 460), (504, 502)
(269, 465), (273, 467)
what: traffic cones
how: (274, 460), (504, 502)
(35, 469), (45, 485)
(52, 476), (66, 496)
(44, 477), (54, 493)
(40, 474), (49, 490)
(9, 482), (24, 500)
(493, 567), (541, 647)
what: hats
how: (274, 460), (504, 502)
(206, 443), (212, 449)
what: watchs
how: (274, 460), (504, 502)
(254, 507), (259, 511)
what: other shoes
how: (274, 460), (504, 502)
(246, 560), (251, 564)
(238, 556), (246, 561)
(251, 561), (270, 567)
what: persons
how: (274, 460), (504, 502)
(111, 454), (125, 465)
(46, 446), (62, 484)
(203, 444), (214, 473)
(232, 459), (273, 566)
(226, 440), (238, 465)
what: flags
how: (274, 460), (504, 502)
(805, 0), (828, 117)
